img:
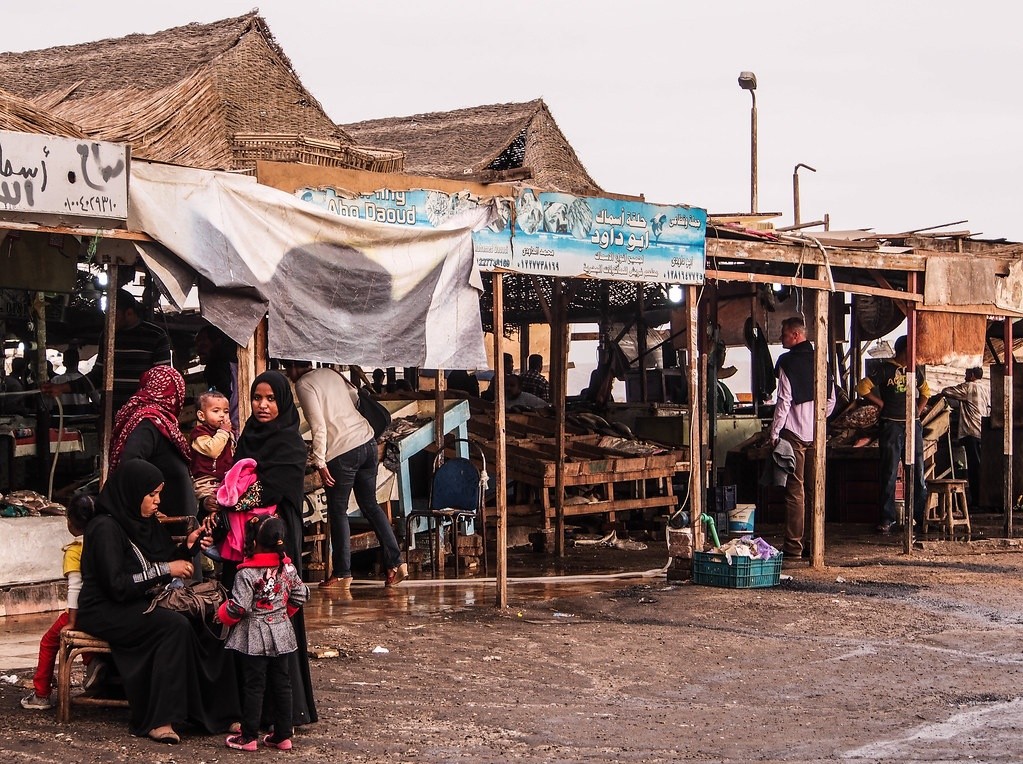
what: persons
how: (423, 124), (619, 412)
(447, 370), (480, 398)
(105, 363), (204, 579)
(75, 461), (242, 743)
(39, 288), (171, 452)
(943, 367), (991, 511)
(19, 493), (100, 707)
(481, 352), (550, 409)
(855, 337), (941, 532)
(761, 318), (837, 559)
(196, 327), (236, 397)
(193, 370), (319, 727)
(188, 391), (238, 564)
(1, 349), (100, 411)
(211, 514), (309, 750)
(385, 379), (413, 392)
(281, 358), (410, 589)
(362, 369), (386, 392)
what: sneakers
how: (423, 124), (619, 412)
(82, 656), (105, 690)
(20, 691), (51, 709)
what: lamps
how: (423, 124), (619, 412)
(182, 356), (206, 376)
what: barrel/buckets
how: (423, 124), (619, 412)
(728, 504), (756, 533)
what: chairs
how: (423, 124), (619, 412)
(406, 439), (487, 580)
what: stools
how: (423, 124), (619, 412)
(923, 479), (972, 536)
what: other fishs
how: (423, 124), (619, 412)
(511, 405), (634, 437)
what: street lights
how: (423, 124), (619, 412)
(737, 70), (759, 214)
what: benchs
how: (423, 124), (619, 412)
(56, 629), (129, 722)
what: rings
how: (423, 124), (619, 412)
(206, 516), (210, 519)
(186, 566), (192, 570)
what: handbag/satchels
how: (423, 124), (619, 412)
(146, 577), (231, 641)
(328, 366), (391, 439)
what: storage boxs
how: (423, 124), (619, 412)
(671, 481), (738, 537)
(692, 548), (784, 590)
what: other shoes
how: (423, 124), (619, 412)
(778, 548), (802, 559)
(225, 734), (258, 751)
(915, 524), (938, 531)
(875, 520), (895, 532)
(203, 546), (221, 561)
(229, 722), (242, 733)
(263, 735), (293, 750)
(149, 728), (180, 744)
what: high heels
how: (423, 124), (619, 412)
(384, 563), (408, 586)
(318, 575), (354, 589)
